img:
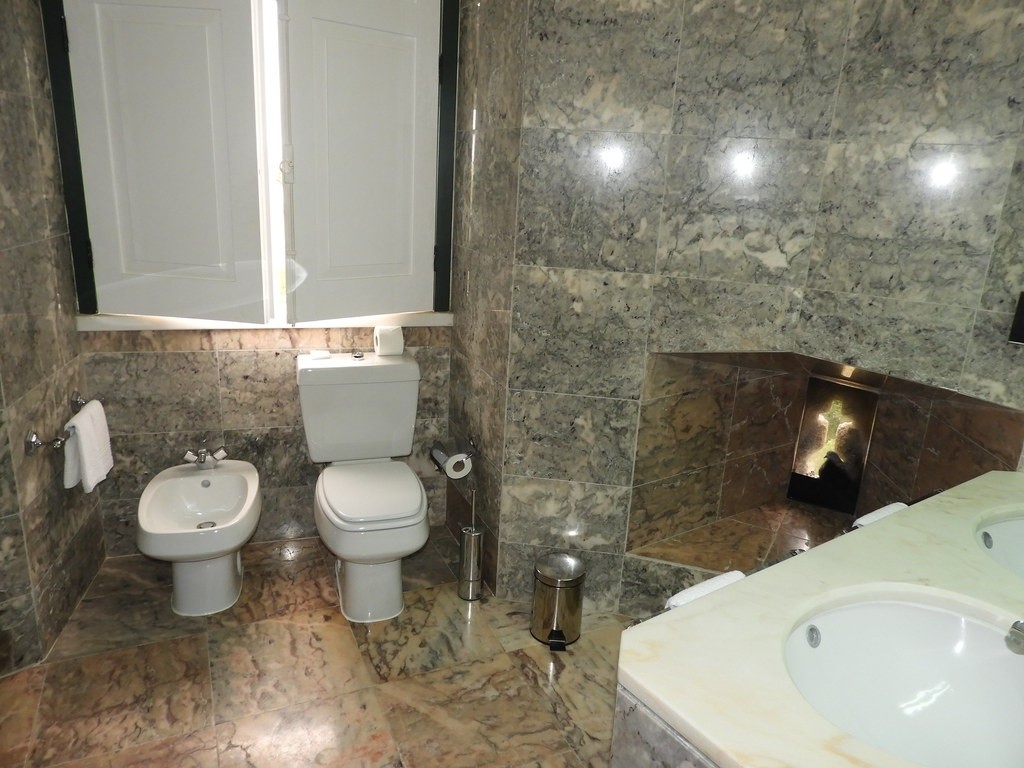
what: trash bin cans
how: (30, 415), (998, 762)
(529, 552), (587, 651)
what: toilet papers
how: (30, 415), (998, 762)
(441, 453), (473, 480)
(373, 325), (404, 356)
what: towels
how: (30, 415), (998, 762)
(664, 570), (746, 610)
(851, 502), (909, 528)
(63, 399), (114, 494)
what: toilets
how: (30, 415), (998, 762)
(296, 351), (430, 624)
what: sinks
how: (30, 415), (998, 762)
(135, 458), (263, 617)
(781, 581), (1023, 768)
(971, 503), (1024, 578)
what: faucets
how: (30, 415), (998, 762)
(180, 446), (229, 470)
(1004, 618), (1023, 655)
